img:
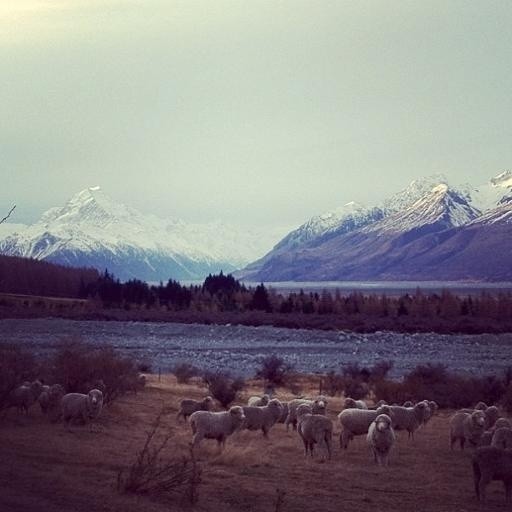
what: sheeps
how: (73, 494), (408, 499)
(176, 394), (438, 466)
(0, 379), (104, 427)
(449, 401), (512, 505)
